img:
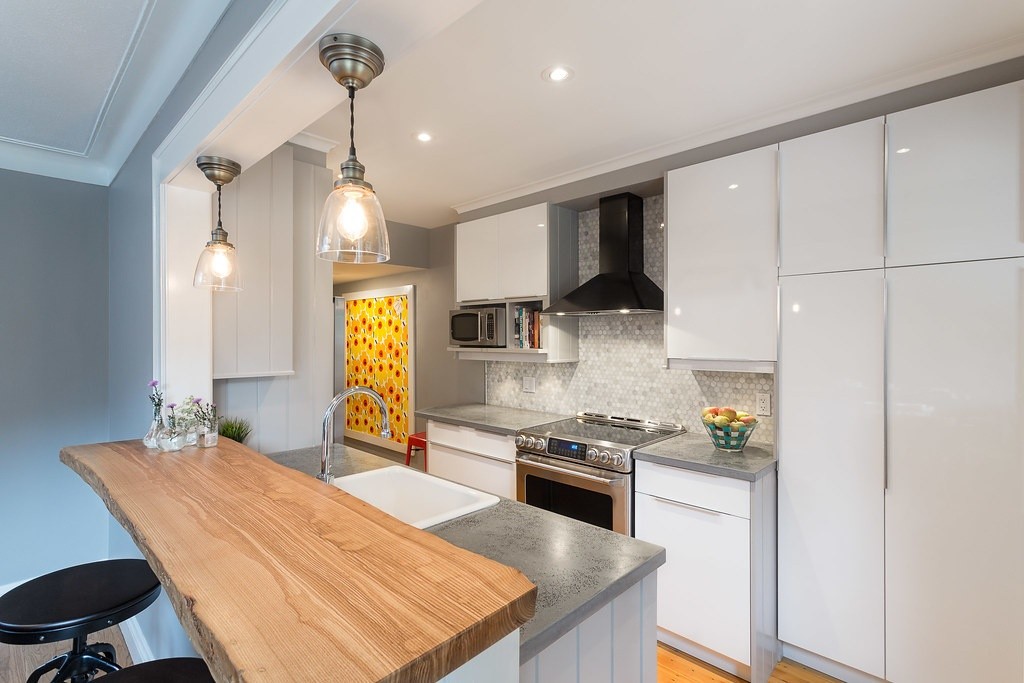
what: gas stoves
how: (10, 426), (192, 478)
(515, 411), (686, 474)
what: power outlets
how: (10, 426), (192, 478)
(756, 392), (771, 415)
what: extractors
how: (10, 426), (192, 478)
(539, 192), (665, 317)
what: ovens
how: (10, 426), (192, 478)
(514, 448), (635, 538)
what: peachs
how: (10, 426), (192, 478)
(702, 405), (757, 434)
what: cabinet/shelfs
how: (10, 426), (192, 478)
(635, 460), (784, 683)
(425, 418), (517, 502)
(446, 201), (580, 363)
(662, 80), (1023, 683)
(518, 570), (657, 683)
(212, 146), (296, 380)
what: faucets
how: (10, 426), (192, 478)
(315, 385), (391, 486)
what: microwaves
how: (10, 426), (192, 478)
(448, 307), (506, 348)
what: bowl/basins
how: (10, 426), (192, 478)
(700, 417), (758, 453)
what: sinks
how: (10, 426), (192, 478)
(334, 464), (500, 531)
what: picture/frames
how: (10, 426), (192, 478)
(341, 284), (416, 456)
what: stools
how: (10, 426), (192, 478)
(0, 558), (215, 683)
(405, 431), (426, 472)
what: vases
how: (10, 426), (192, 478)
(143, 405), (165, 448)
(155, 409), (218, 452)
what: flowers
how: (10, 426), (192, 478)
(166, 403), (182, 441)
(146, 380), (164, 424)
(177, 397), (224, 434)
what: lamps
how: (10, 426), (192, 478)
(192, 156), (245, 292)
(316, 33), (390, 264)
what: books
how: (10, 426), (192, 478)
(514, 305), (542, 348)
